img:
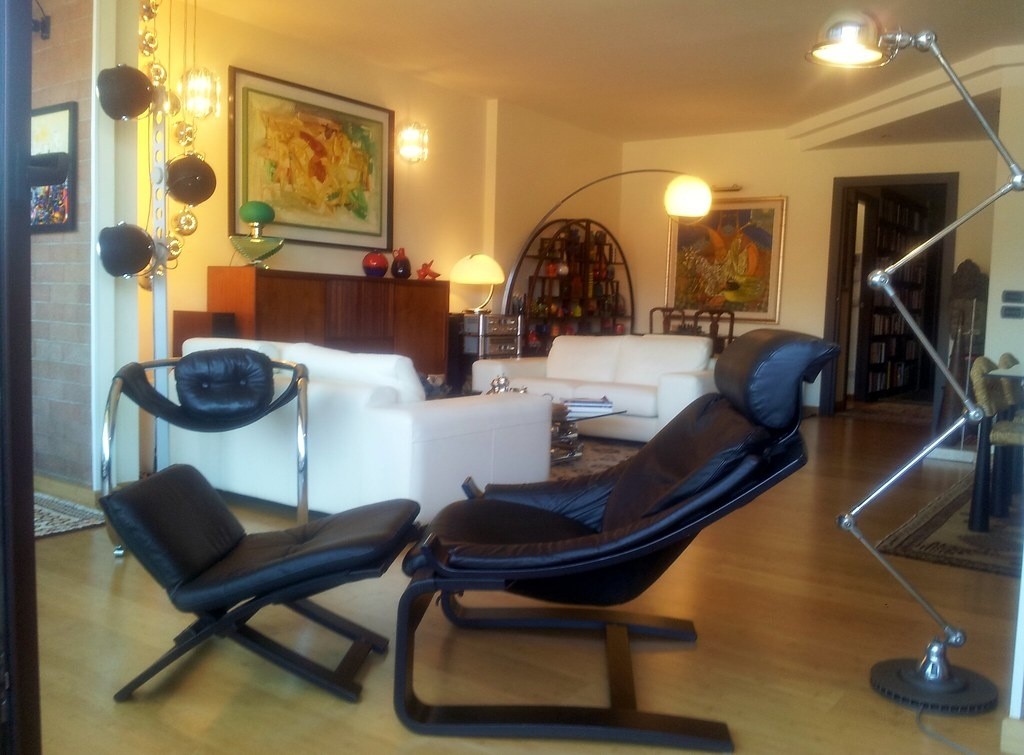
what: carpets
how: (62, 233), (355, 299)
(34, 492), (107, 538)
(875, 466), (1024, 581)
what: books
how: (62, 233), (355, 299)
(562, 396), (613, 412)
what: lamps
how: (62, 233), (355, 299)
(801, 6), (1024, 718)
(97, 3), (220, 478)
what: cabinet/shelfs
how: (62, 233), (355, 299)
(504, 218), (633, 361)
(857, 198), (935, 393)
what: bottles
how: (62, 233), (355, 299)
(362, 252), (388, 277)
(546, 261), (557, 276)
(607, 265), (615, 280)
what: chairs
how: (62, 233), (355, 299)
(969, 352), (1024, 536)
(99, 349), (304, 557)
(392, 328), (838, 751)
(102, 461), (421, 705)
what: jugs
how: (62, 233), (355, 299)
(391, 248), (411, 278)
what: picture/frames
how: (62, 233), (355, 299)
(228, 65), (395, 252)
(664, 195), (788, 324)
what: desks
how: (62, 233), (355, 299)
(550, 402), (628, 466)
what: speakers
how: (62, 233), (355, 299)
(172, 311), (237, 358)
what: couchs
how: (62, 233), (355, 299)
(152, 340), (549, 528)
(472, 334), (721, 446)
